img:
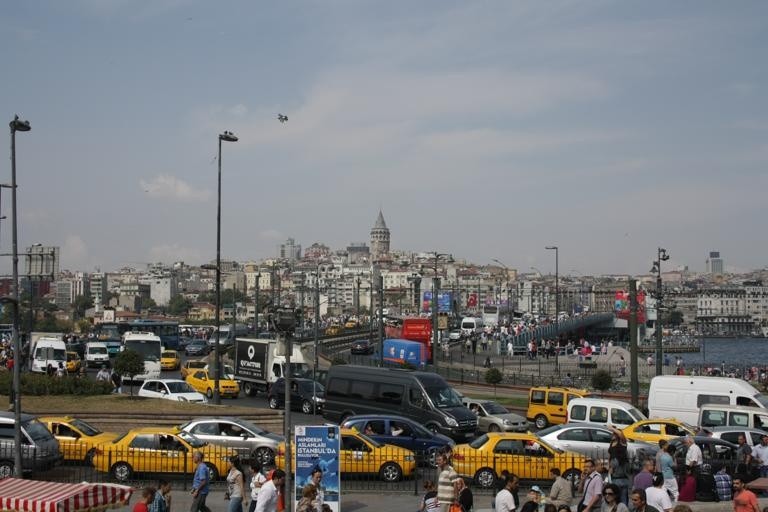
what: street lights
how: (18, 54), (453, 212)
(230, 250), (376, 415)
(528, 265), (546, 315)
(492, 258), (510, 312)
(644, 246), (672, 374)
(542, 245), (563, 374)
(418, 249), (454, 375)
(6, 112), (31, 480)
(200, 129), (239, 406)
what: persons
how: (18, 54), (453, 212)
(131, 425), (333, 512)
(421, 423), (768, 512)
(183, 327), (213, 341)
(472, 405), (479, 415)
(2, 333), (123, 394)
(291, 383), (299, 392)
(442, 312), (626, 385)
(647, 355), (768, 384)
(365, 423), (404, 437)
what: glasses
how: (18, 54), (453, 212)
(603, 491), (615, 496)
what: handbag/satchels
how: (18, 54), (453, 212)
(223, 491), (231, 501)
(576, 498), (585, 512)
